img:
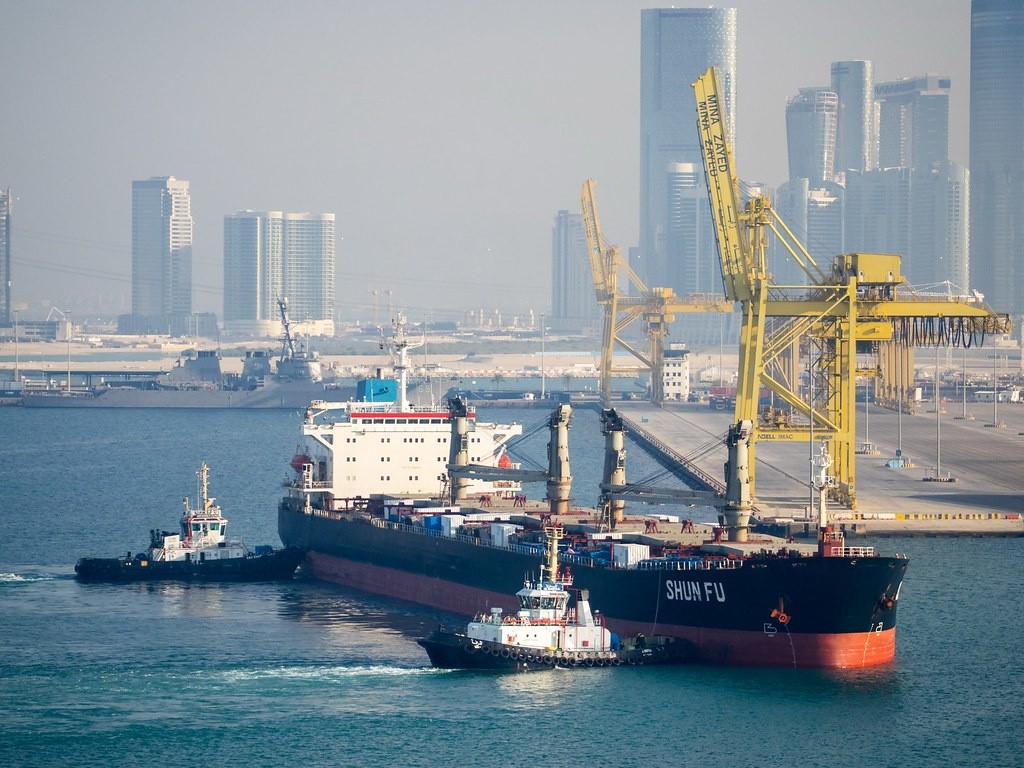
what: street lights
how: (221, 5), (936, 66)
(193, 309), (200, 360)
(539, 312), (546, 399)
(64, 307), (73, 395)
(714, 303), (726, 387)
(305, 311), (309, 352)
(369, 288), (393, 326)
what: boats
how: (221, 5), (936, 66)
(417, 519), (701, 669)
(74, 461), (310, 583)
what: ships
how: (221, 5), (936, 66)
(277, 311), (910, 669)
(22, 297), (358, 407)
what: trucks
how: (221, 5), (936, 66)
(709, 386), (780, 410)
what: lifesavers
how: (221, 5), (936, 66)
(464, 645), (679, 666)
